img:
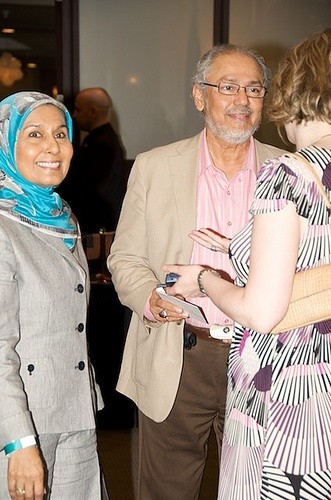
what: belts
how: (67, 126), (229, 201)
(188, 324), (234, 341)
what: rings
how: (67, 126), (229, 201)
(159, 310), (168, 317)
(211, 245), (217, 252)
(166, 317), (168, 321)
(16, 488), (25, 496)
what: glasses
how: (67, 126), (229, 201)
(200, 80), (268, 99)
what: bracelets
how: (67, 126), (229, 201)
(198, 269), (222, 296)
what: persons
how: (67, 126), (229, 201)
(163, 28), (331, 500)
(60, 87), (124, 235)
(0, 92), (108, 500)
(106, 44), (292, 500)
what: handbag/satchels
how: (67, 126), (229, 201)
(269, 152), (331, 334)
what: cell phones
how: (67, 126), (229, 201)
(157, 272), (180, 288)
